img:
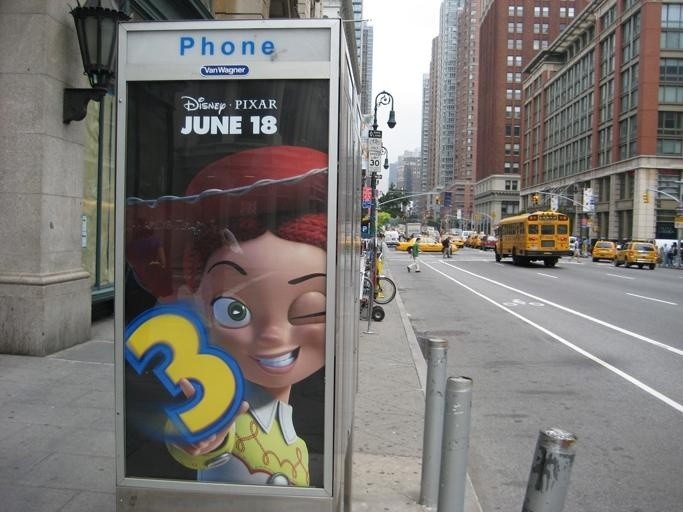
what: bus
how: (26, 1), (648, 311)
(495, 209), (570, 266)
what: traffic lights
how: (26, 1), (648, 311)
(532, 195), (539, 205)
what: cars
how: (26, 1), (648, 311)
(592, 241), (657, 270)
(396, 229), (498, 254)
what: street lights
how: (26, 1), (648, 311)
(371, 89), (395, 270)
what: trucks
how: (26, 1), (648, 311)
(406, 223), (422, 238)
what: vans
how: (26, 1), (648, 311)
(384, 231), (399, 245)
(570, 236), (578, 255)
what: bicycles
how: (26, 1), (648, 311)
(364, 276), (396, 304)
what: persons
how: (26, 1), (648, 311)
(443, 238), (452, 258)
(656, 242), (683, 268)
(125, 148), (328, 487)
(407, 238), (421, 272)
(569, 239), (582, 263)
